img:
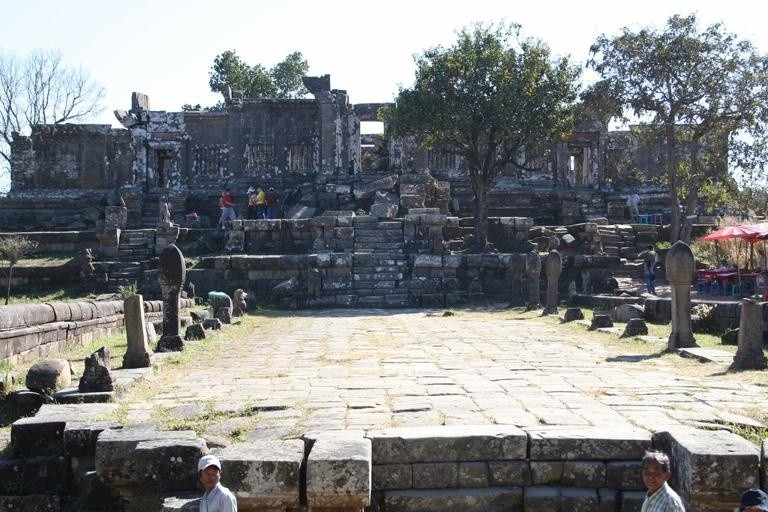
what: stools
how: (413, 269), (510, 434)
(696, 267), (768, 299)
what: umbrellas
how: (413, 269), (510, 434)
(697, 224), (761, 287)
(741, 222), (768, 274)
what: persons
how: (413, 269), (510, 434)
(192, 453), (239, 511)
(641, 244), (657, 295)
(219, 188), (237, 225)
(264, 187), (280, 220)
(246, 186), (258, 220)
(218, 191), (226, 220)
(87, 248), (96, 271)
(81, 248), (96, 279)
(185, 207), (200, 228)
(252, 186), (265, 220)
(754, 246), (768, 303)
(619, 189), (641, 224)
(637, 451), (684, 512)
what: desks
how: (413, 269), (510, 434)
(635, 213), (663, 224)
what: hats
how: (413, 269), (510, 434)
(195, 454), (223, 474)
